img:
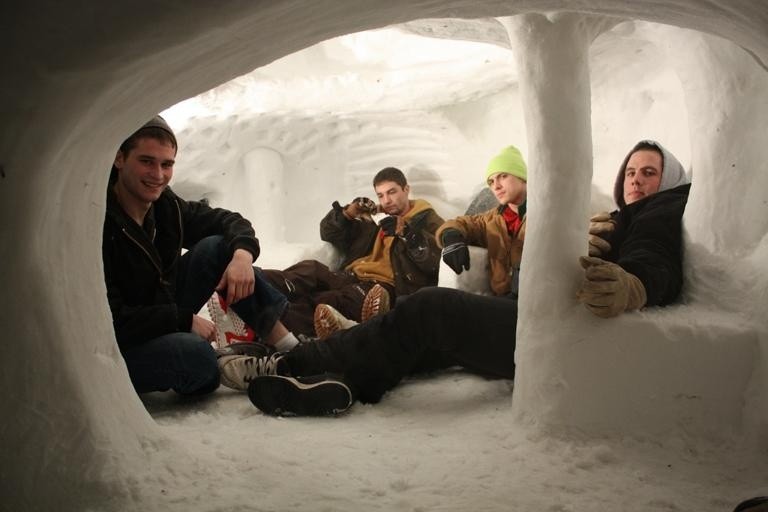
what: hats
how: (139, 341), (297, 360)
(143, 115), (178, 157)
(486, 145), (526, 186)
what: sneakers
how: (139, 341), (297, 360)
(248, 373), (357, 417)
(207, 291), (255, 354)
(314, 303), (360, 341)
(361, 283), (391, 321)
(217, 334), (317, 393)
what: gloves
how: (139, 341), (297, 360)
(578, 255), (648, 319)
(377, 215), (414, 242)
(588, 211), (618, 256)
(343, 197), (377, 224)
(442, 230), (469, 274)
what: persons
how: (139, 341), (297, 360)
(314, 145), (528, 337)
(102, 114), (315, 402)
(214, 138), (693, 416)
(206, 167), (445, 349)
